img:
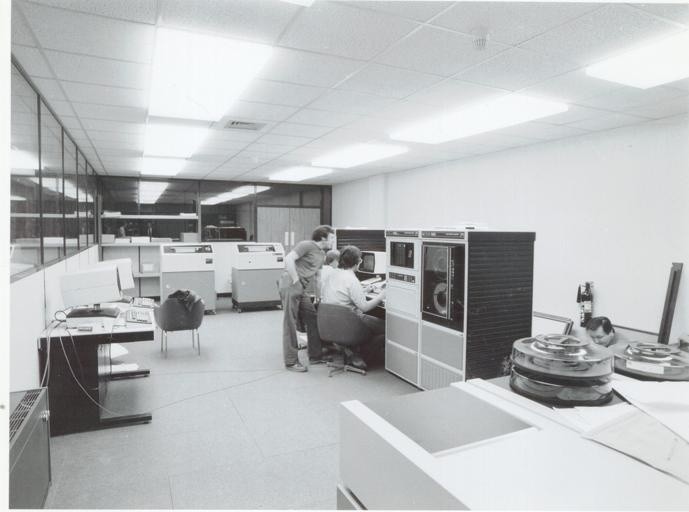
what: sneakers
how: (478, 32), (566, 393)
(287, 363), (307, 371)
(353, 356), (367, 370)
(310, 353), (333, 364)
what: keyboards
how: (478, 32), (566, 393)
(133, 297), (153, 308)
(126, 308), (152, 324)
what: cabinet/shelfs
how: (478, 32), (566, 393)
(100, 214), (200, 299)
(10, 213), (95, 249)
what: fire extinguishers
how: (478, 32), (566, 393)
(577, 280), (595, 327)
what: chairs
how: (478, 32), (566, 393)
(316, 302), (375, 378)
(153, 296), (206, 356)
(290, 294), (322, 349)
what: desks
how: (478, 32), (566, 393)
(38, 297), (155, 437)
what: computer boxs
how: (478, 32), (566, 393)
(9, 385), (52, 508)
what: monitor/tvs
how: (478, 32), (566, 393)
(60, 263), (123, 317)
(79, 258), (135, 290)
(358, 250), (386, 277)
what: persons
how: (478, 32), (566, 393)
(315, 249), (340, 304)
(585, 315), (630, 350)
(275, 224), (340, 373)
(326, 244), (386, 371)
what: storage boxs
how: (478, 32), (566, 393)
(11, 246), (78, 268)
